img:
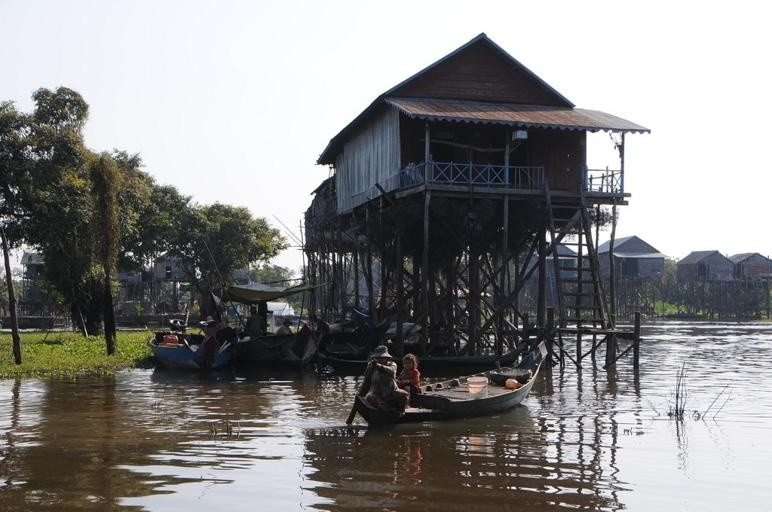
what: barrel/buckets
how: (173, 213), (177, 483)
(162, 334), (178, 346)
(467, 376), (489, 400)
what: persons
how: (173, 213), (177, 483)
(397, 353), (420, 409)
(309, 315), (329, 333)
(277, 319), (292, 335)
(357, 345), (408, 418)
(246, 307), (265, 337)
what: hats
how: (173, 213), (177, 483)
(368, 345), (392, 358)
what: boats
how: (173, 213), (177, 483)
(318, 321), (535, 375)
(149, 325), (320, 382)
(355, 341), (548, 423)
